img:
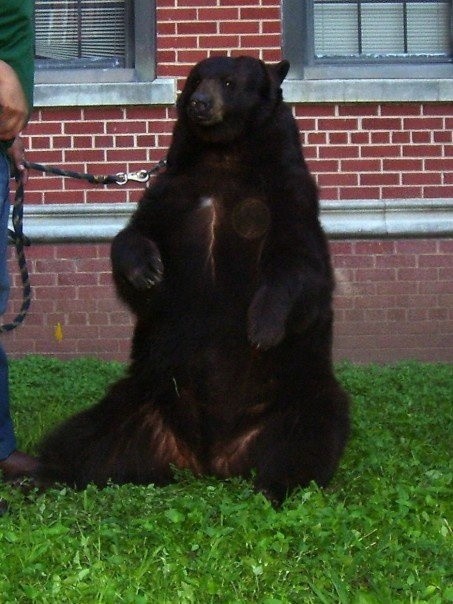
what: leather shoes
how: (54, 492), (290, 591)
(1, 451), (43, 480)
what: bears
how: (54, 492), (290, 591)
(24, 53), (356, 517)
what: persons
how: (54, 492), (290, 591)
(1, 1), (50, 481)
